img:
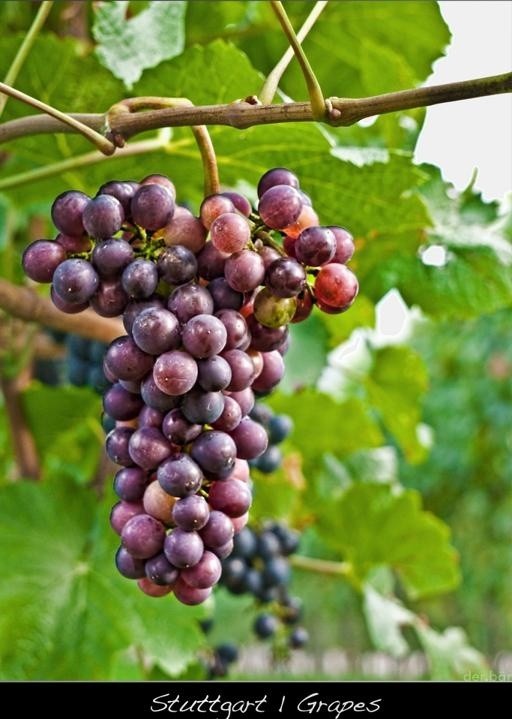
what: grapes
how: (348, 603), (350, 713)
(21, 167), (361, 682)
